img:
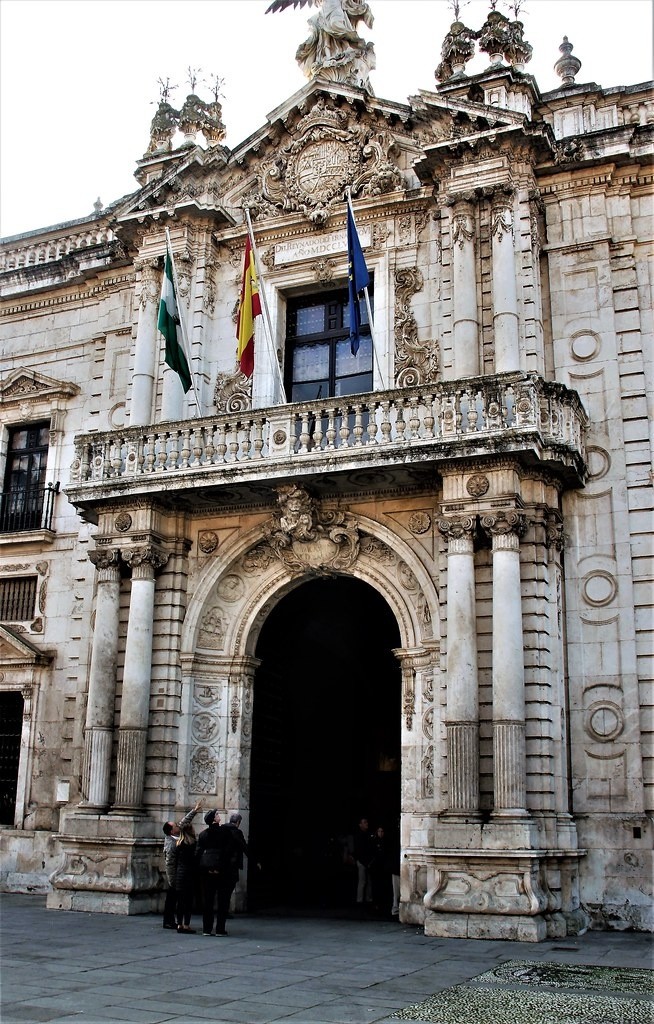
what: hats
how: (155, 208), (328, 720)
(204, 808), (217, 825)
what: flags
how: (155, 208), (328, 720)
(347, 202), (369, 358)
(236, 234), (262, 380)
(158, 246), (192, 394)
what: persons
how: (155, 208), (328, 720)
(307, 0), (360, 57)
(163, 805), (261, 937)
(350, 818), (399, 916)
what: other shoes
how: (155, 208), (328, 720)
(203, 933), (211, 936)
(216, 931), (228, 936)
(163, 924), (178, 929)
(177, 928), (196, 934)
(392, 907), (399, 915)
(226, 913), (234, 919)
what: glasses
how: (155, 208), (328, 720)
(174, 822), (180, 828)
